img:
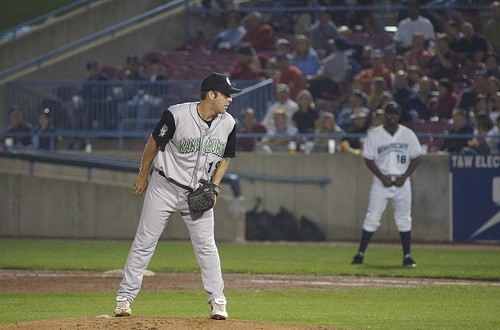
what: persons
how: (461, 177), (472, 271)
(351, 102), (422, 267)
(0, 0), (500, 154)
(115, 72), (242, 320)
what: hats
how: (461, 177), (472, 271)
(277, 84), (289, 94)
(346, 90), (369, 101)
(434, 32), (452, 45)
(200, 71), (241, 97)
(385, 102), (400, 113)
(370, 48), (385, 60)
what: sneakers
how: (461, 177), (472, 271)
(208, 298), (228, 320)
(114, 296), (131, 316)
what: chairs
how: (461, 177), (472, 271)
(105, 37), (269, 99)
(356, 30), (465, 146)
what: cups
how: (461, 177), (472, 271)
(328, 139), (335, 153)
(288, 141), (296, 153)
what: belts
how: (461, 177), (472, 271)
(158, 170), (193, 193)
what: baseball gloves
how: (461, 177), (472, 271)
(187, 186), (218, 214)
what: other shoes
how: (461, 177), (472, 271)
(403, 257), (416, 267)
(353, 255), (362, 265)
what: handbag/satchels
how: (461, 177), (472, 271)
(298, 217), (324, 241)
(244, 198), (271, 241)
(271, 206), (296, 240)
